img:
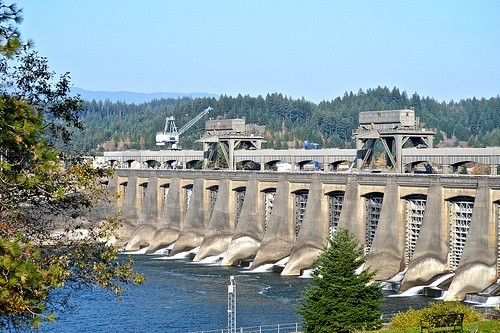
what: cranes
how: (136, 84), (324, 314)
(155, 106), (213, 150)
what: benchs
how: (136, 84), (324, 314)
(420, 313), (466, 333)
